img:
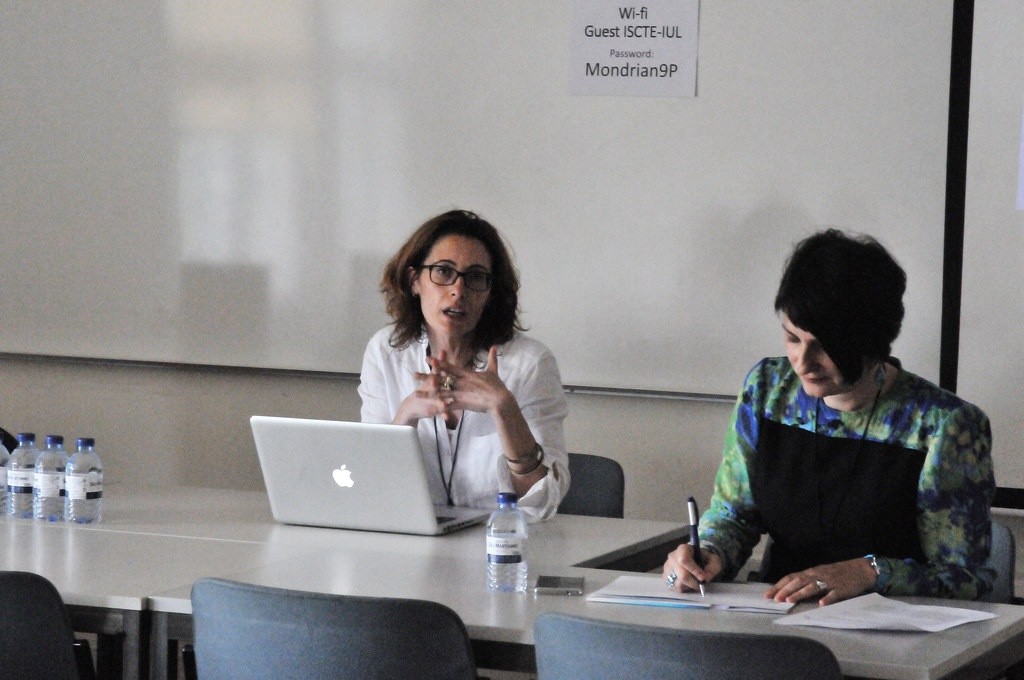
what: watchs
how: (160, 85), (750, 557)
(866, 554), (891, 596)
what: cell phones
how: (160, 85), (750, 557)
(535, 575), (585, 596)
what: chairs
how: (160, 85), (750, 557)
(557, 453), (623, 520)
(183, 575), (489, 679)
(533, 612), (845, 680)
(0, 571), (98, 680)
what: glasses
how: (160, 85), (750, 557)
(414, 263), (496, 292)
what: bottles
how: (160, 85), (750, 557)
(0, 433), (10, 518)
(7, 433), (40, 518)
(65, 438), (103, 525)
(33, 435), (70, 523)
(486, 493), (527, 593)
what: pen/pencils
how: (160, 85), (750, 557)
(686, 496), (707, 597)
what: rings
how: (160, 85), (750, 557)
(443, 373), (458, 389)
(666, 573), (678, 588)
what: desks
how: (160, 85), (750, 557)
(0, 488), (1024, 680)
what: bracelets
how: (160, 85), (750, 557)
(505, 443), (546, 477)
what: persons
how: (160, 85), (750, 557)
(660, 224), (1001, 606)
(357, 209), (571, 523)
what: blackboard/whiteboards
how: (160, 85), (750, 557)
(2, 0), (972, 403)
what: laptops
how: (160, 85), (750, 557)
(250, 415), (496, 535)
